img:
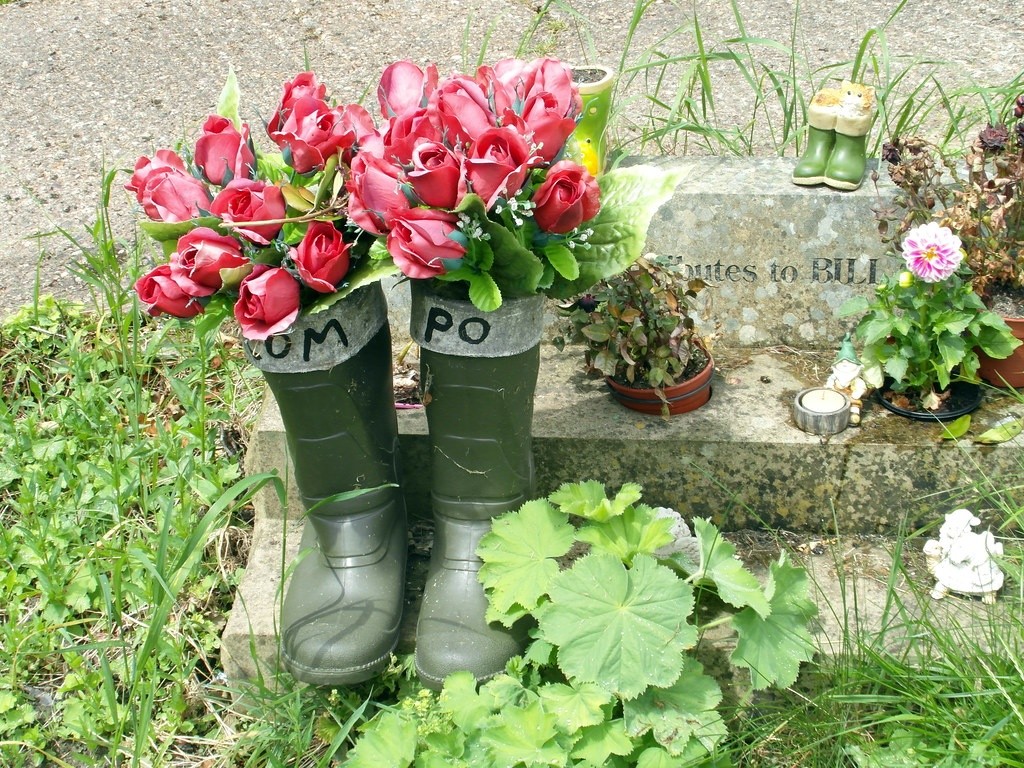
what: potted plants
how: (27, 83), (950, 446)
(569, 251), (721, 416)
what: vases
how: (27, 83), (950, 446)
(972, 311), (1024, 389)
(874, 380), (998, 423)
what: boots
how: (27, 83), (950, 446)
(410, 281), (549, 691)
(239, 283), (407, 685)
(826, 106), (873, 192)
(793, 99), (837, 185)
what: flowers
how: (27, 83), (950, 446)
(883, 93), (1024, 300)
(123, 50), (697, 344)
(841, 214), (1014, 387)
